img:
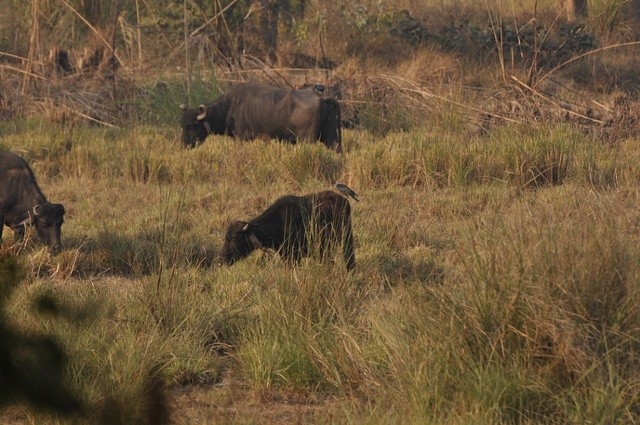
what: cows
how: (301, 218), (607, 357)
(0, 149), (65, 257)
(218, 190), (354, 270)
(177, 81), (342, 151)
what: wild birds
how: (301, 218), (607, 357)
(331, 180), (361, 203)
